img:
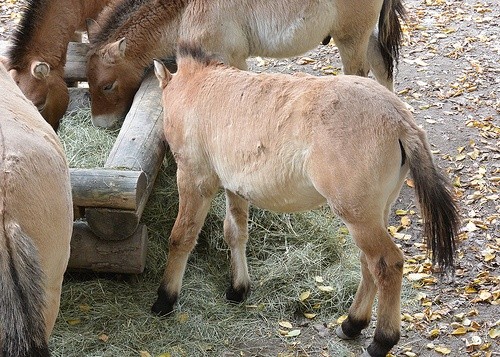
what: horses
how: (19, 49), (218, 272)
(6, 0), (115, 134)
(0, 59), (73, 357)
(152, 42), (462, 357)
(86, 0), (409, 128)
